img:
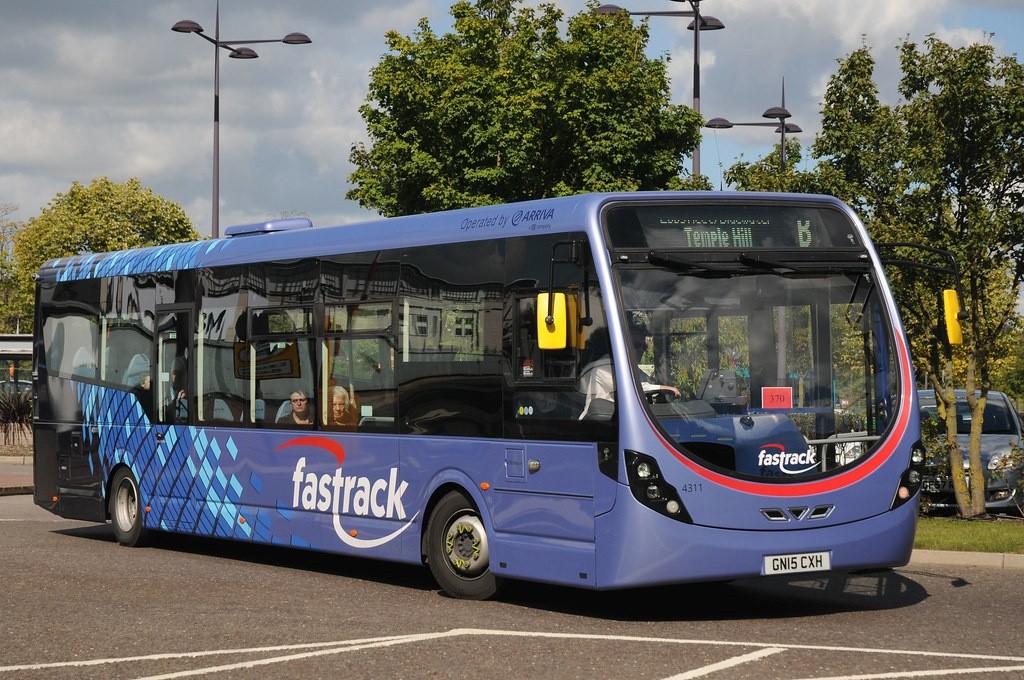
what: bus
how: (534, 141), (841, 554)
(26, 187), (969, 602)
(0, 331), (35, 405)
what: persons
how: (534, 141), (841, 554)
(326, 385), (358, 426)
(278, 383), (314, 425)
(570, 307), (681, 417)
(134, 371), (185, 424)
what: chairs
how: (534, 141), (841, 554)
(169, 357), (186, 382)
(255, 399), (266, 422)
(203, 399), (234, 423)
(165, 399), (190, 425)
(275, 400), (293, 424)
(125, 354), (150, 388)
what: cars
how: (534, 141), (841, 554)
(0, 380), (32, 397)
(914, 389), (1024, 518)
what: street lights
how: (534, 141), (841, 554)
(703, 108), (802, 194)
(595, 0), (727, 187)
(170, 20), (314, 240)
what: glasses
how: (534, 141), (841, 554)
(633, 340), (649, 350)
(291, 399), (305, 403)
